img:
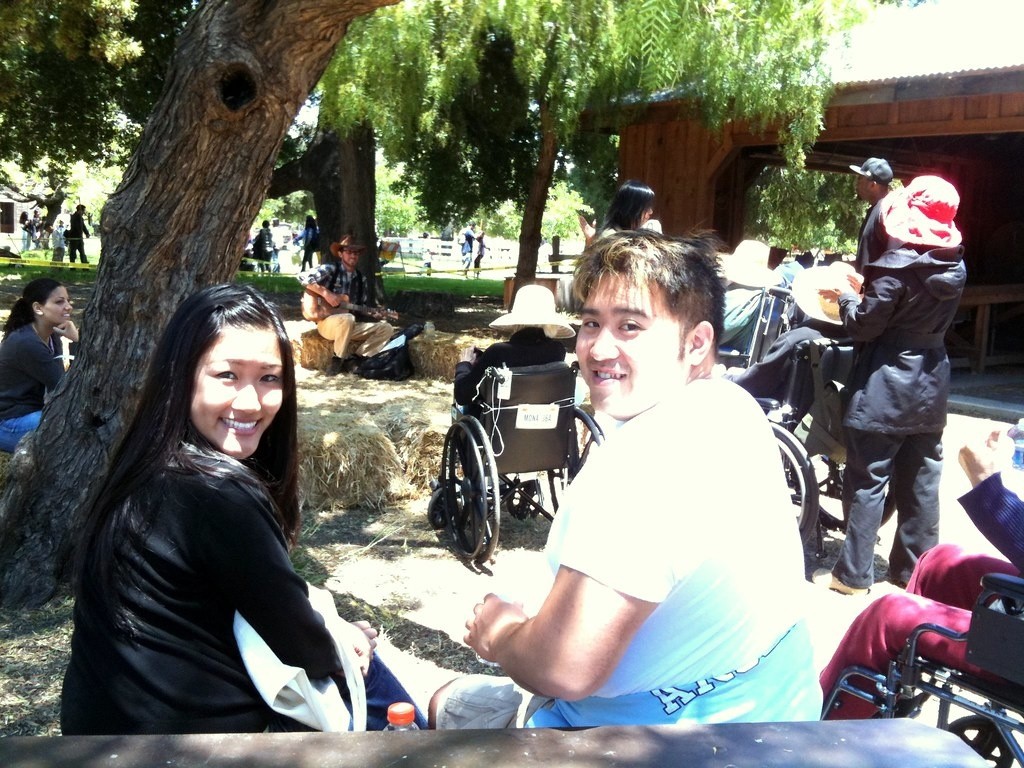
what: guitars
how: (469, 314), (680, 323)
(301, 288), (399, 324)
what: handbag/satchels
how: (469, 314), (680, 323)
(232, 582), (368, 731)
(359, 344), (416, 382)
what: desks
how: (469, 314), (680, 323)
(0, 716), (993, 768)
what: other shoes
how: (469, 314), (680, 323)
(811, 567), (869, 595)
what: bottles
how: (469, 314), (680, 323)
(1007, 418), (1024, 480)
(382, 701), (420, 731)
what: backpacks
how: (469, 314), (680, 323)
(458, 228), (467, 245)
(309, 228), (321, 251)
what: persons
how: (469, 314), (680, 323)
(60, 283), (424, 735)
(43, 222), (65, 262)
(292, 216), (320, 271)
(70, 204), (90, 264)
(20, 210), (47, 249)
(577, 182), (672, 249)
(0, 277), (81, 452)
(421, 232), (434, 269)
(822, 405), (1024, 722)
(701, 155), (966, 596)
(256, 218), (274, 274)
(305, 235), (394, 378)
(455, 217), (488, 280)
(447, 284), (579, 420)
(429, 230), (824, 725)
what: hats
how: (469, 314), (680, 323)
(716, 239), (784, 288)
(880, 174), (962, 247)
(850, 157), (893, 185)
(330, 235), (367, 257)
(489, 285), (576, 339)
(792, 259), (863, 326)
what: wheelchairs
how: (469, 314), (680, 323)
(822, 577), (1024, 768)
(427, 358), (606, 562)
(713, 339), (898, 547)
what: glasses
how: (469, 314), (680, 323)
(340, 249), (361, 257)
